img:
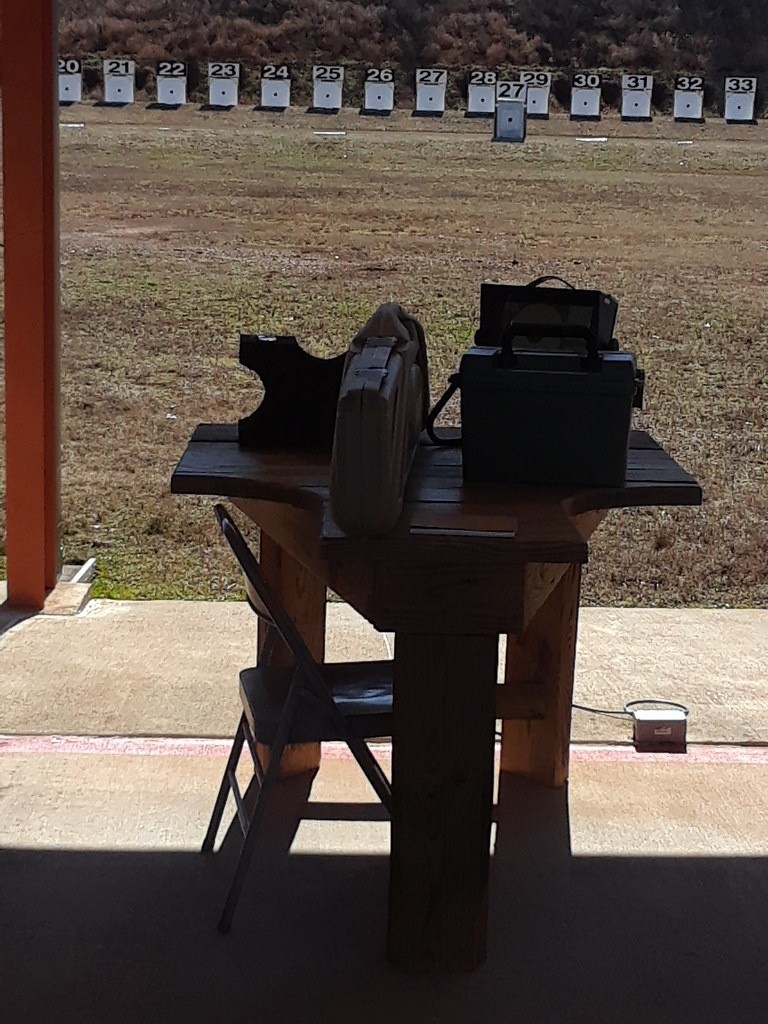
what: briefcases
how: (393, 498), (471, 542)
(328, 301), (431, 540)
(459, 320), (645, 489)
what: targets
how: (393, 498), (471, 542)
(158, 76), (186, 107)
(725, 91), (755, 120)
(497, 106), (526, 141)
(310, 80), (344, 110)
(262, 79), (290, 109)
(570, 88), (600, 116)
(364, 81), (394, 112)
(522, 85), (550, 116)
(102, 75), (135, 104)
(416, 84), (444, 113)
(57, 74), (84, 103)
(623, 88), (651, 120)
(467, 84), (495, 115)
(672, 90), (703, 120)
(209, 78), (239, 108)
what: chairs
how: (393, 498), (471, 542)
(196, 505), (393, 936)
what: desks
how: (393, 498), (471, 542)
(170, 424), (703, 978)
